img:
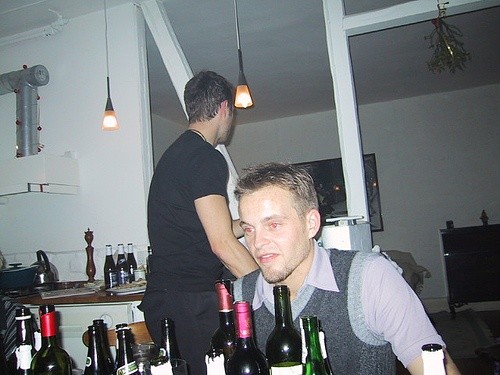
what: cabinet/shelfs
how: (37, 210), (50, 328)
(440, 224), (500, 319)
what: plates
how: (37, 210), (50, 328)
(106, 281), (147, 295)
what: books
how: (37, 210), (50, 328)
(41, 287), (95, 299)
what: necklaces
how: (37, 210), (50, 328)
(189, 129), (206, 142)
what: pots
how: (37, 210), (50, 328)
(1, 263), (39, 290)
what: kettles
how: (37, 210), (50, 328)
(29, 249), (60, 286)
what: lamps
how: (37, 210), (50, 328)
(232, 0), (253, 109)
(102, 0), (117, 131)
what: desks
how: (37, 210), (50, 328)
(8, 280), (144, 304)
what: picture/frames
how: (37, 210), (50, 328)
(288, 154), (383, 232)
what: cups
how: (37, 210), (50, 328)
(134, 270), (146, 281)
(170, 358), (188, 375)
(130, 343), (157, 375)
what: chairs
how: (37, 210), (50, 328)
(83, 321), (153, 347)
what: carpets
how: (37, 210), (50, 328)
(435, 308), (495, 360)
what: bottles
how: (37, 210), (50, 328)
(226, 300), (271, 375)
(266, 284), (305, 375)
(421, 343), (446, 375)
(299, 315), (334, 375)
(7, 308), (37, 375)
(83, 318), (179, 375)
(146, 246), (153, 277)
(104, 243), (137, 290)
(209, 278), (236, 375)
(30, 304), (72, 375)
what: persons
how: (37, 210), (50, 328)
(229, 162), (463, 375)
(142, 71), (260, 375)
